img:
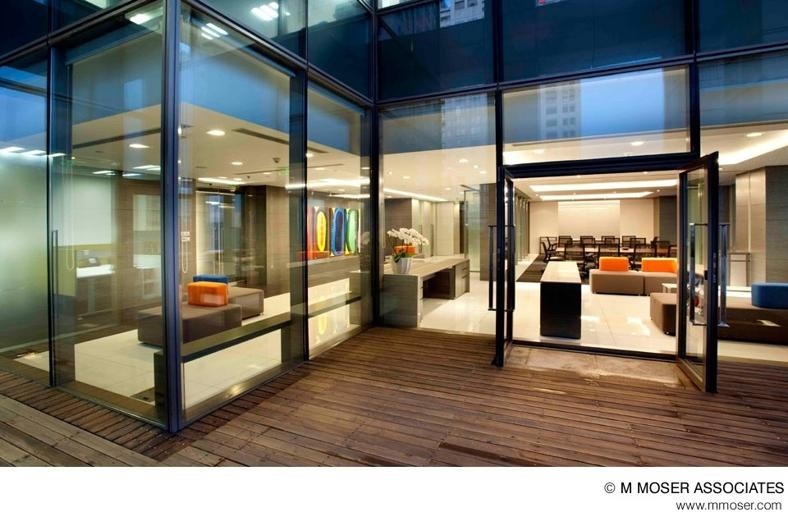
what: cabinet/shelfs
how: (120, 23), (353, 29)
(75, 264), (116, 329)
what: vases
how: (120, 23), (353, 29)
(390, 255), (414, 274)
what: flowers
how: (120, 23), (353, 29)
(385, 227), (428, 257)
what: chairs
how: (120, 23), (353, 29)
(540, 234), (678, 271)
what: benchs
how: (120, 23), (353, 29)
(648, 283), (787, 344)
(588, 256), (645, 296)
(136, 281), (240, 348)
(192, 274), (266, 319)
(637, 258), (678, 295)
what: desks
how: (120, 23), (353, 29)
(348, 256), (470, 328)
(660, 282), (677, 292)
(539, 260), (583, 340)
